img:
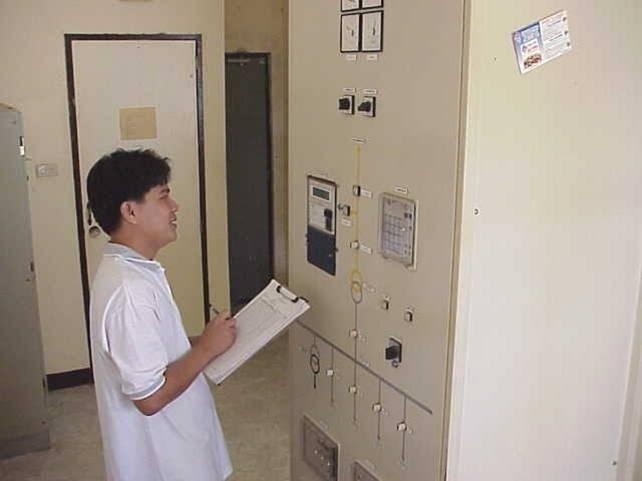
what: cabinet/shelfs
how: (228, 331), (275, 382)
(1, 103), (53, 460)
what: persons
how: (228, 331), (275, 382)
(85, 147), (241, 481)
